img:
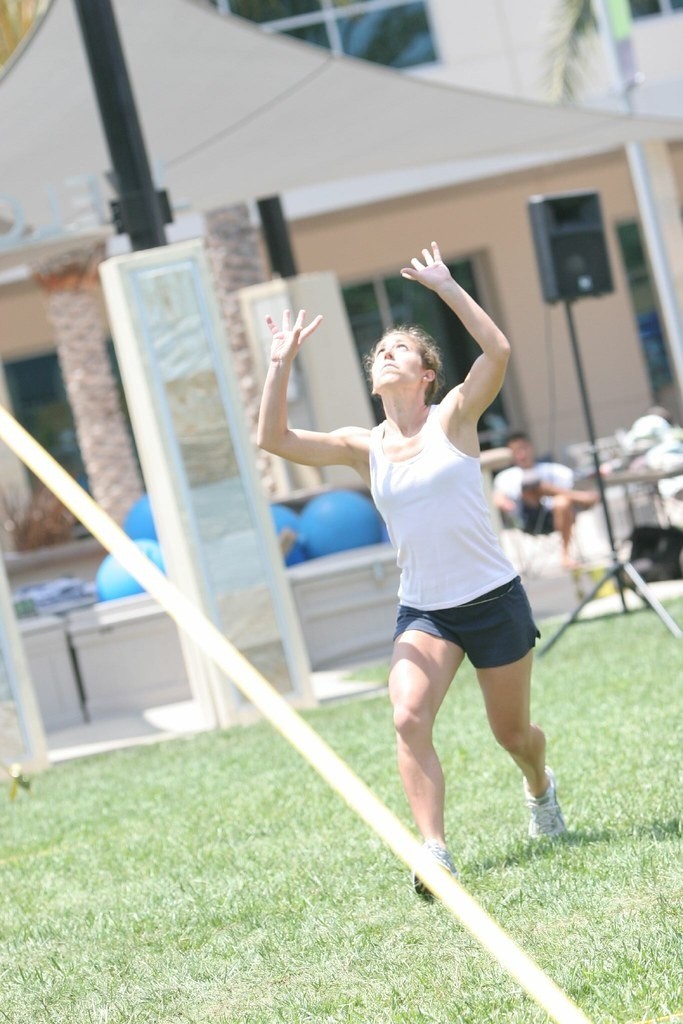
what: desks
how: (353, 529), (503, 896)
(32, 593), (100, 726)
(585, 463), (683, 584)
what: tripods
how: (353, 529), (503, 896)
(534, 302), (683, 664)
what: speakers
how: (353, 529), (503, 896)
(530, 189), (616, 306)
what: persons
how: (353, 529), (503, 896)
(256, 240), (571, 904)
(493, 431), (599, 570)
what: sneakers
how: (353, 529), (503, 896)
(411, 840), (459, 902)
(524, 765), (565, 841)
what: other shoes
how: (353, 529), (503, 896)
(561, 560), (583, 571)
(574, 490), (602, 513)
